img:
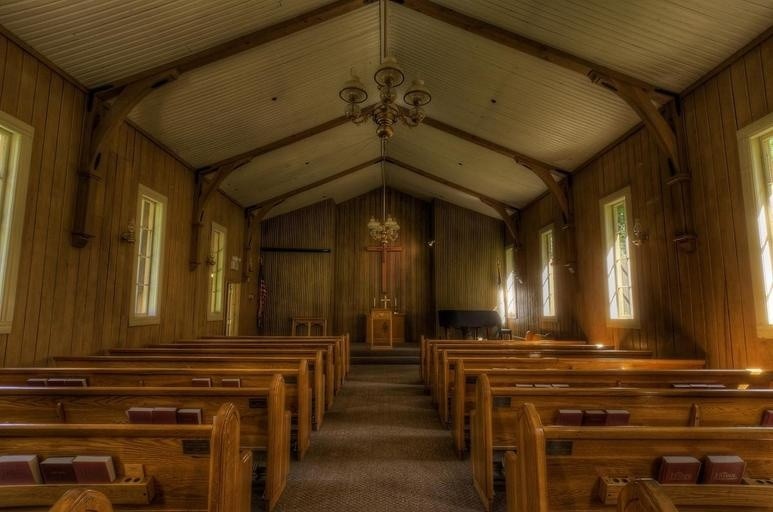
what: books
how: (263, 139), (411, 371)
(175, 407), (202, 425)
(604, 409), (630, 426)
(761, 407), (773, 427)
(127, 406), (155, 424)
(582, 409), (606, 426)
(151, 406), (177, 424)
(70, 453), (117, 484)
(25, 377), (88, 387)
(37, 454), (79, 484)
(671, 382), (726, 389)
(555, 408), (585, 425)
(220, 377), (241, 387)
(0, 452), (43, 485)
(190, 377), (213, 388)
(513, 382), (570, 388)
(699, 455), (745, 485)
(657, 455), (702, 484)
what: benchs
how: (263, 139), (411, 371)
(421, 330), (773, 512)
(0, 333), (351, 512)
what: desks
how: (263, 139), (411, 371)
(438, 310), (501, 339)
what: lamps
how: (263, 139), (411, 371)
(338, 0), (432, 145)
(630, 218), (649, 247)
(121, 219), (136, 243)
(368, 161), (401, 248)
